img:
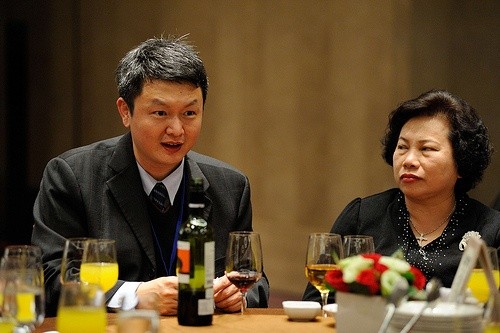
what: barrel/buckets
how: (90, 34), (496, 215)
(385, 301), (485, 333)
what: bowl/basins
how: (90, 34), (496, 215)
(283, 300), (322, 321)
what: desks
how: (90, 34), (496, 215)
(35, 308), (335, 333)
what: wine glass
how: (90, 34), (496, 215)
(225, 231), (266, 321)
(304, 232), (346, 310)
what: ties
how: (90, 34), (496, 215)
(151, 181), (168, 212)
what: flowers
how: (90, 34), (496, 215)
(322, 247), (426, 305)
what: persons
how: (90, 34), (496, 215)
(32, 37), (269, 315)
(303, 90), (500, 310)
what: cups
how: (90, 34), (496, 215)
(323, 304), (337, 327)
(466, 247), (500, 305)
(55, 237), (119, 333)
(116, 310), (159, 333)
(0, 245), (45, 333)
(344, 236), (374, 258)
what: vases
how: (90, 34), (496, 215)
(335, 291), (387, 333)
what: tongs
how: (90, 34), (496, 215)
(377, 278), (440, 333)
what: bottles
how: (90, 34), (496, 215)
(177, 177), (216, 324)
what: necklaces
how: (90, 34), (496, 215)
(409, 202), (455, 245)
